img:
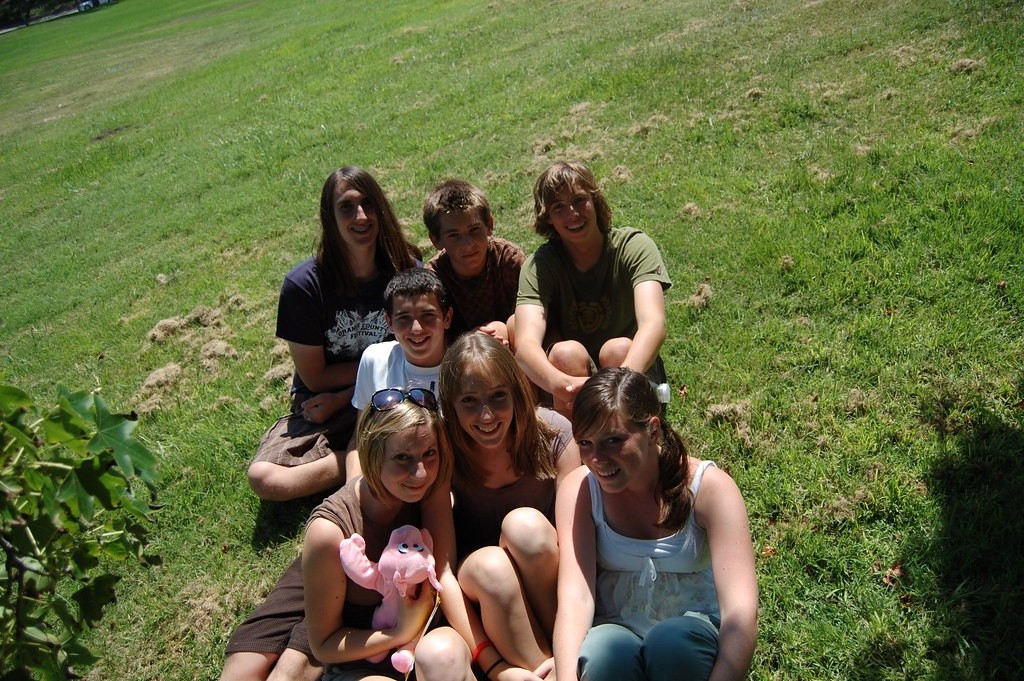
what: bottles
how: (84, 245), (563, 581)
(649, 380), (671, 404)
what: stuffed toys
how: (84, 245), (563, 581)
(339, 524), (443, 673)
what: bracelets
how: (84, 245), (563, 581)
(483, 658), (502, 679)
(473, 640), (491, 663)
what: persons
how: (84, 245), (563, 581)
(552, 368), (757, 681)
(219, 159), (673, 681)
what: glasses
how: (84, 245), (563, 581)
(372, 388), (439, 411)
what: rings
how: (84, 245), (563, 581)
(305, 416), (310, 422)
(432, 591), (436, 595)
